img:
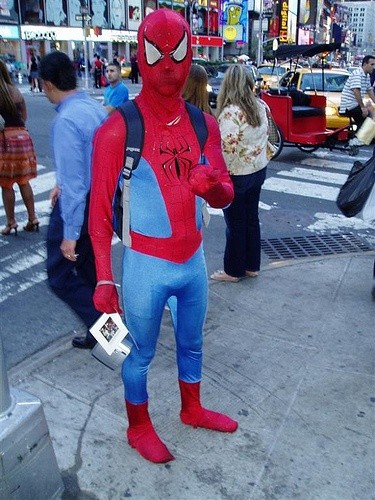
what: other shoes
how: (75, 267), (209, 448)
(209, 269), (241, 283)
(72, 331), (98, 350)
(246, 269), (258, 277)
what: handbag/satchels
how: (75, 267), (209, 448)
(335, 156), (375, 227)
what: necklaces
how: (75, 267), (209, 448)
(140, 90), (184, 126)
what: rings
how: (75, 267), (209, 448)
(64, 254), (68, 257)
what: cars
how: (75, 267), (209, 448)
(119, 60), (132, 79)
(78, 57), (92, 72)
(277, 67), (355, 128)
(191, 55), (367, 109)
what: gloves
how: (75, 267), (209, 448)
(93, 284), (124, 314)
(188, 165), (223, 202)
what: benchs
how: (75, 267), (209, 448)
(266, 86), (324, 118)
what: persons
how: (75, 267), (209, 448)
(77, 52), (140, 89)
(181, 63), (211, 114)
(340, 55), (375, 127)
(211, 64), (276, 281)
(41, 51), (123, 349)
(87, 11), (238, 464)
(4, 55), (42, 92)
(0, 58), (40, 235)
(101, 61), (129, 115)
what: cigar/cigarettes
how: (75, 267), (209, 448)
(67, 254), (79, 257)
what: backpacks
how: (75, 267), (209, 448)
(111, 99), (209, 241)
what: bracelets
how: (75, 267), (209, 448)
(97, 280), (120, 287)
(361, 107), (366, 110)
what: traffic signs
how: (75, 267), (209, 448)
(75, 14), (92, 22)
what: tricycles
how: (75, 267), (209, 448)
(260, 42), (375, 161)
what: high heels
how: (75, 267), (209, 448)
(0, 221), (19, 235)
(23, 218), (40, 231)
